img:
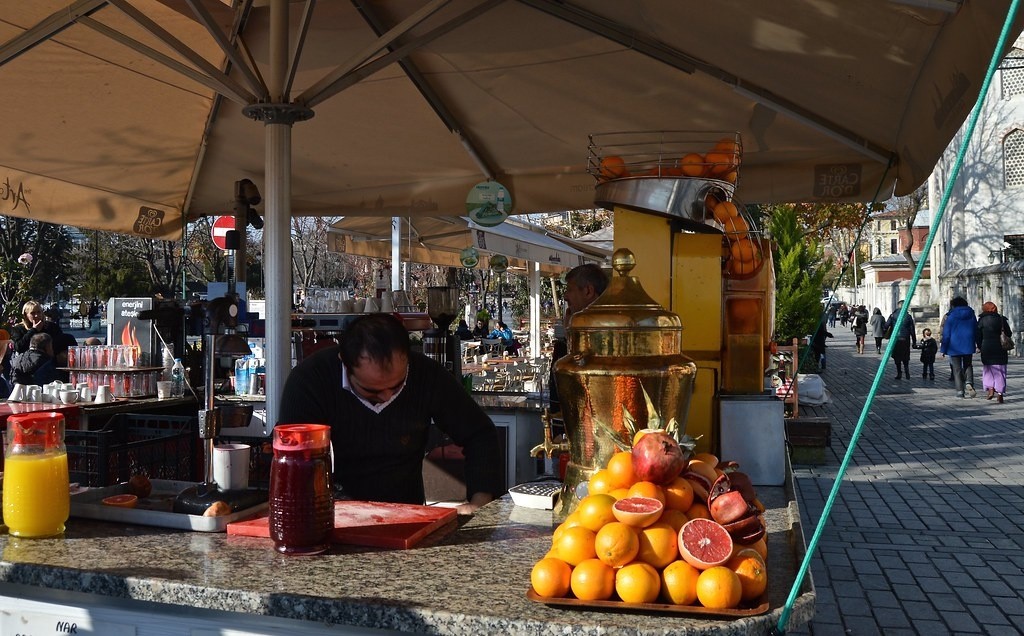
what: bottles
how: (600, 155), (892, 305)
(257, 372), (265, 395)
(173, 359), (184, 398)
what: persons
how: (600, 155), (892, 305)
(454, 318), (513, 356)
(547, 262), (609, 420)
(6, 299), (102, 392)
(827, 295), (1013, 403)
(278, 313), (505, 512)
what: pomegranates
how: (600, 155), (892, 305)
(633, 432), (684, 485)
(708, 474), (765, 543)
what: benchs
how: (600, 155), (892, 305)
(785, 401), (833, 447)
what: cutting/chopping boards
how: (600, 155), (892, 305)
(227, 500), (457, 550)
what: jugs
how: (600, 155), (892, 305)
(269, 424), (335, 557)
(3, 412), (69, 540)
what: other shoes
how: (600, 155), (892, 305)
(930, 375), (934, 380)
(922, 374), (927, 379)
(956, 390), (965, 398)
(878, 347), (880, 354)
(905, 374), (910, 380)
(966, 384), (976, 398)
(895, 374), (902, 379)
(997, 394), (1004, 403)
(987, 388), (994, 400)
(949, 377), (954, 381)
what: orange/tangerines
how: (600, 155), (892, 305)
(531, 428), (767, 608)
(723, 299), (768, 392)
(601, 138), (761, 272)
(102, 495), (137, 506)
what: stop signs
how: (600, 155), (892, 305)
(211, 215), (235, 250)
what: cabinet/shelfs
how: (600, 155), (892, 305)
(612, 204), (781, 455)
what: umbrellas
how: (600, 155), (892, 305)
(1, 1), (1022, 434)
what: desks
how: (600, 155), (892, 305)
(0, 398), (203, 489)
(461, 341), (481, 365)
(485, 357), (524, 383)
(524, 347), (554, 357)
(462, 363), (505, 391)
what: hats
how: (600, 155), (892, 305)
(982, 302), (997, 313)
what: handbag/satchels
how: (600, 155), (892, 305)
(1000, 314), (1015, 351)
(882, 316), (887, 333)
(851, 317), (857, 327)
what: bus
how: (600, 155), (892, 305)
(820, 297), (836, 304)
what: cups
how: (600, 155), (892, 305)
(304, 287), (350, 313)
(94, 384), (115, 404)
(81, 387), (91, 401)
(8, 384), (26, 401)
(76, 383), (88, 401)
(354, 302), (364, 313)
(212, 443), (251, 490)
(67, 345), (157, 397)
(157, 381), (172, 399)
(42, 380), (63, 404)
(365, 297), (379, 313)
(393, 290), (405, 312)
(32, 389), (42, 402)
(59, 390), (79, 405)
(380, 291), (393, 313)
(61, 384), (73, 391)
(26, 385), (41, 400)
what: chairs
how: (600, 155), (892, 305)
(461, 332), (558, 390)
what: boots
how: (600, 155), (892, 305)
(857, 344), (864, 354)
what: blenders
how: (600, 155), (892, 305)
(420, 285), (459, 374)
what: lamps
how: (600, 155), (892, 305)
(988, 248), (1004, 264)
(225, 179), (264, 251)
(215, 327), (253, 356)
